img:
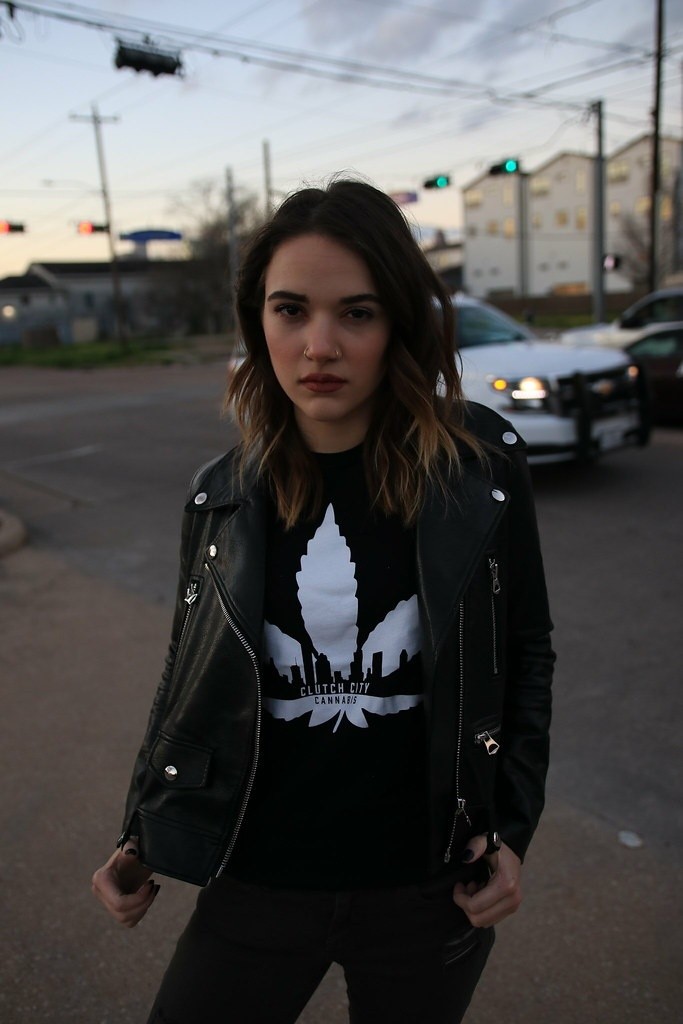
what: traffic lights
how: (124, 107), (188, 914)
(424, 175), (448, 188)
(0, 219), (23, 236)
(490, 160), (518, 174)
(77, 221), (109, 234)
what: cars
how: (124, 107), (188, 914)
(558, 288), (683, 392)
(228, 294), (649, 466)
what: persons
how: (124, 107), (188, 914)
(94, 182), (558, 1024)
